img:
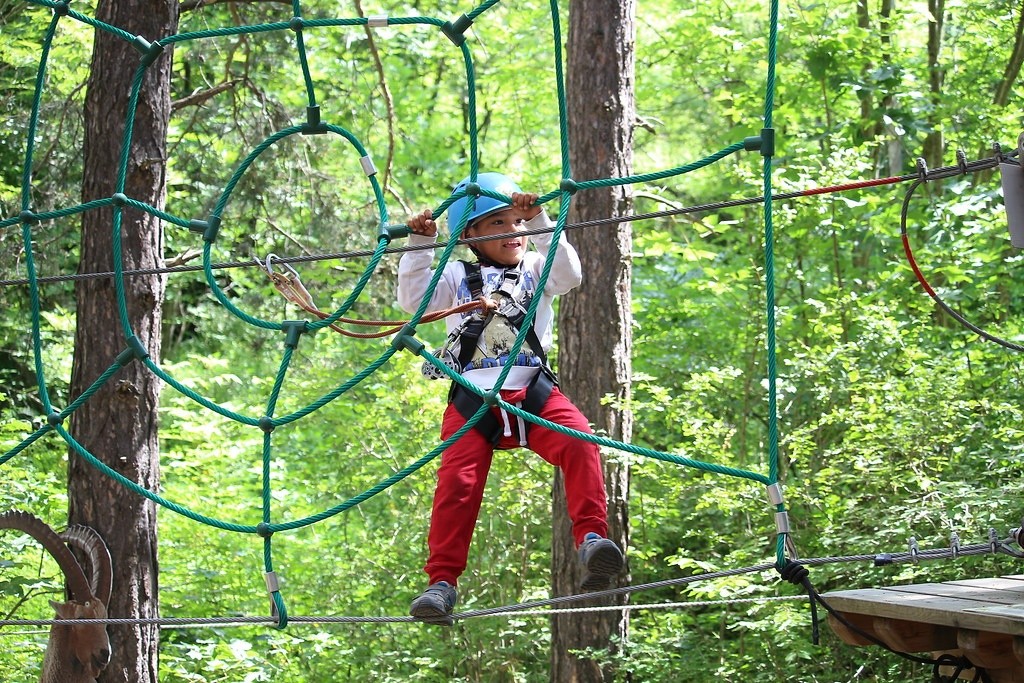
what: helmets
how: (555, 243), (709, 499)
(447, 172), (522, 236)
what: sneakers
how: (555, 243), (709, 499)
(410, 580), (456, 626)
(576, 531), (623, 594)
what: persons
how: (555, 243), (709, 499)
(397, 172), (625, 626)
